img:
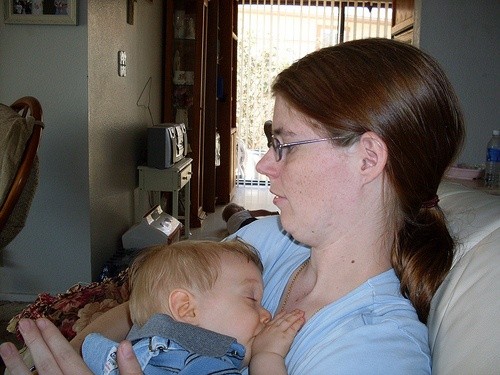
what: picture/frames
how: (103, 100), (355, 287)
(3, 0), (80, 26)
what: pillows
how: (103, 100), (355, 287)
(7, 268), (129, 342)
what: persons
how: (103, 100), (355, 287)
(1, 37), (467, 375)
(222, 201), (282, 234)
(82, 235), (305, 375)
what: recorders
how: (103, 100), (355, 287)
(146, 122), (188, 170)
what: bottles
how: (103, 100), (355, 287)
(485, 130), (500, 189)
(185, 13), (195, 40)
(172, 48), (185, 85)
(183, 47), (194, 85)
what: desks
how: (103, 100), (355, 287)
(136, 158), (194, 243)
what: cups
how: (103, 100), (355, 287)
(172, 9), (185, 40)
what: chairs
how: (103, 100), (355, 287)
(0, 95), (45, 249)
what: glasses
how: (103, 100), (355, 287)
(267, 120), (364, 162)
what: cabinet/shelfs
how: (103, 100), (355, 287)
(163, 0), (237, 226)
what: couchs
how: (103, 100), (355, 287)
(428, 179), (500, 374)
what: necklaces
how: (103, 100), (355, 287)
(282, 258), (314, 313)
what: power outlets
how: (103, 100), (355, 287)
(119, 50), (126, 77)
